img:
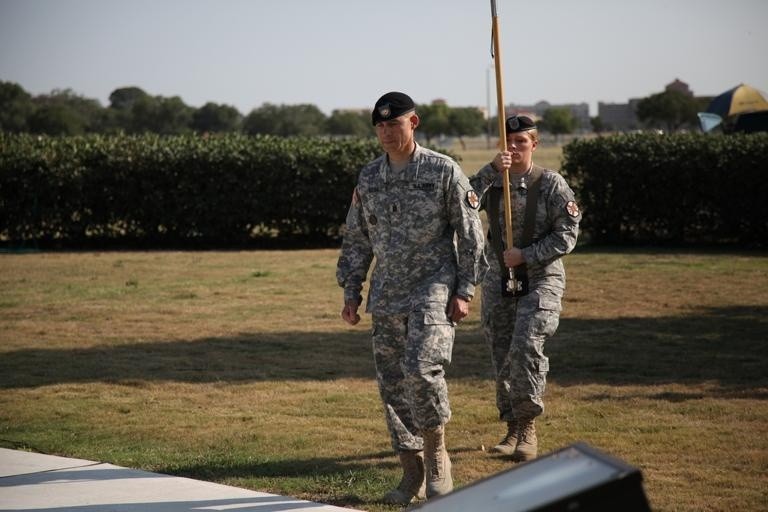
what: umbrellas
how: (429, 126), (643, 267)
(708, 82), (768, 116)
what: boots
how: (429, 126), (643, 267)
(384, 425), (453, 504)
(492, 417), (537, 461)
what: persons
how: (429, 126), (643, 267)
(467, 112), (583, 463)
(335, 91), (492, 507)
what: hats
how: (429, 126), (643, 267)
(506, 116), (537, 133)
(372, 92), (415, 125)
